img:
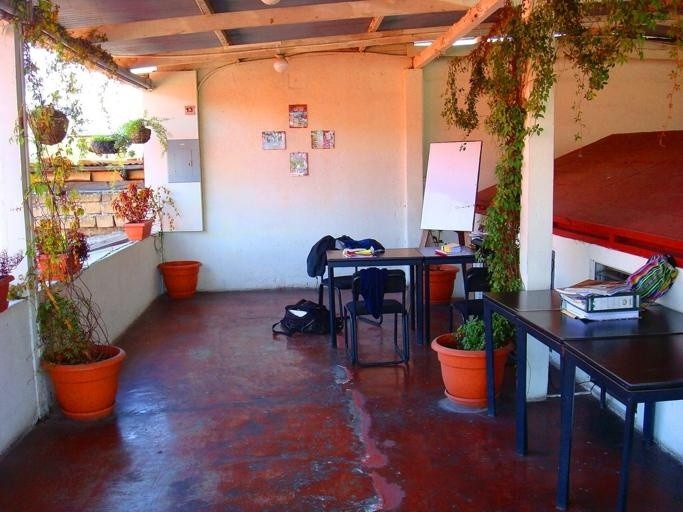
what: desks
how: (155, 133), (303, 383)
(326, 248), (424, 348)
(409, 246), (494, 347)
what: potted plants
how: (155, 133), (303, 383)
(143, 184), (202, 300)
(0, 247), (29, 313)
(7, 100), (91, 279)
(431, 1), (682, 407)
(117, 108), (170, 153)
(76, 133), (131, 194)
(6, 221), (127, 420)
(110, 183), (160, 241)
(422, 230), (459, 306)
(0, 0), (119, 146)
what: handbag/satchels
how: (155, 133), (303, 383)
(281, 299), (331, 336)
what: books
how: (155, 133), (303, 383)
(555, 279), (640, 321)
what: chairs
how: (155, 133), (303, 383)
(344, 269), (410, 367)
(319, 267), (383, 327)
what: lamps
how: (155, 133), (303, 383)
(274, 52), (289, 74)
